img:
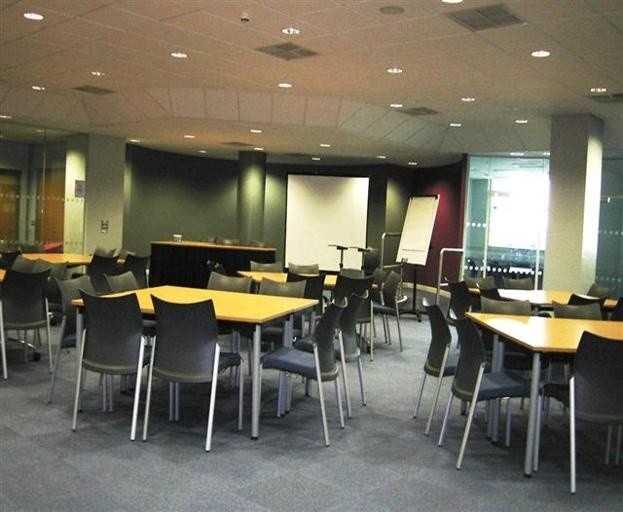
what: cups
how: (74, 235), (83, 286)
(173, 234), (182, 243)
